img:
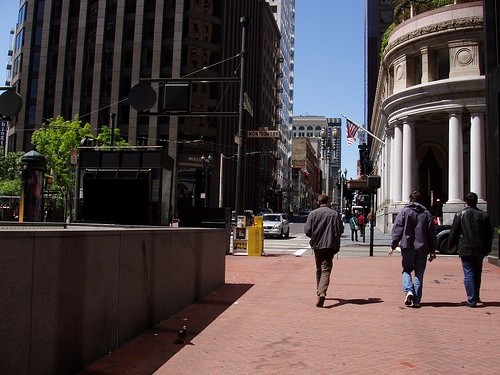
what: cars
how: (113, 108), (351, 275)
(261, 213), (290, 238)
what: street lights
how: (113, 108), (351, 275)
(200, 152), (214, 207)
(338, 168), (348, 213)
(320, 125), (339, 196)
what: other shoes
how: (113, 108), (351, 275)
(316, 296), (325, 307)
(404, 292), (414, 305)
(414, 302), (421, 307)
(462, 300), (477, 308)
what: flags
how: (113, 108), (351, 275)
(346, 119), (359, 147)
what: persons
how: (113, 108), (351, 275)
(448, 192), (493, 308)
(304, 194), (345, 307)
(386, 191), (437, 307)
(350, 212), (365, 241)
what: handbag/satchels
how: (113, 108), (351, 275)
(355, 225), (359, 230)
